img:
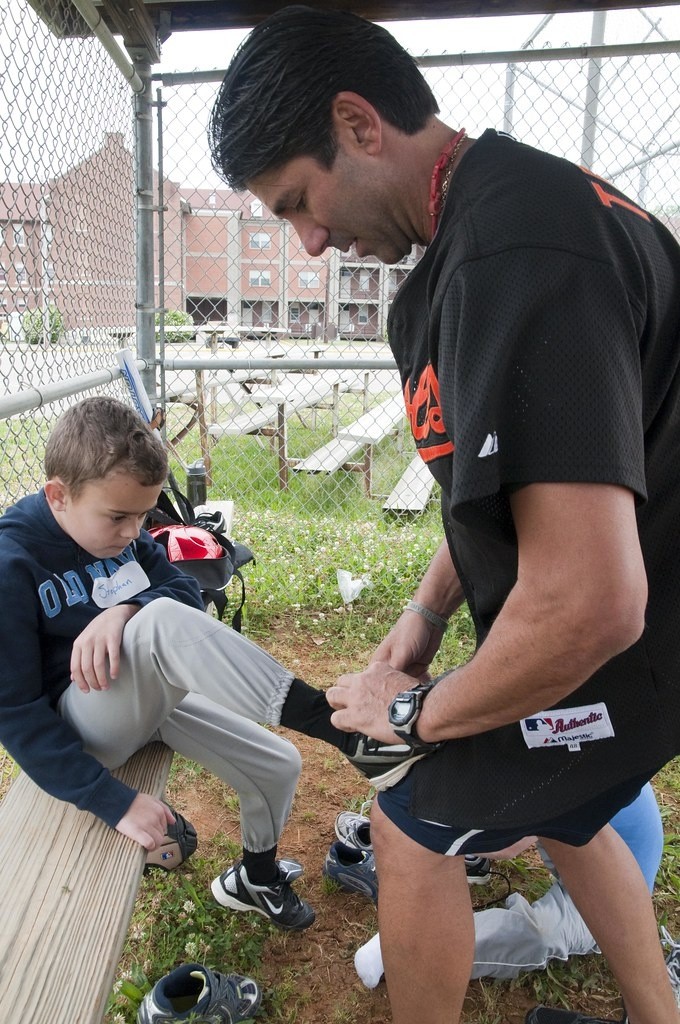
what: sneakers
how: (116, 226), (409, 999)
(660, 925), (680, 1008)
(465, 854), (492, 885)
(527, 1007), (629, 1024)
(334, 800), (372, 850)
(343, 730), (449, 793)
(324, 841), (378, 903)
(211, 859), (316, 931)
(135, 964), (262, 1024)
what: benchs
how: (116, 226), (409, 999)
(206, 337), (240, 349)
(0, 500), (233, 1024)
(153, 370), (436, 512)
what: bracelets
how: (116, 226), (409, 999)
(402, 601), (450, 631)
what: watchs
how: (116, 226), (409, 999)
(387, 683), (443, 750)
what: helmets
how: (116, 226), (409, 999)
(147, 525), (223, 562)
(142, 811), (198, 877)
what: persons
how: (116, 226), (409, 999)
(205, 1), (680, 1024)
(0, 400), (441, 931)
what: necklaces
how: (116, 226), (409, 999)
(429, 129), (467, 236)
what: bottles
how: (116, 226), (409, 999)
(186, 458), (207, 511)
